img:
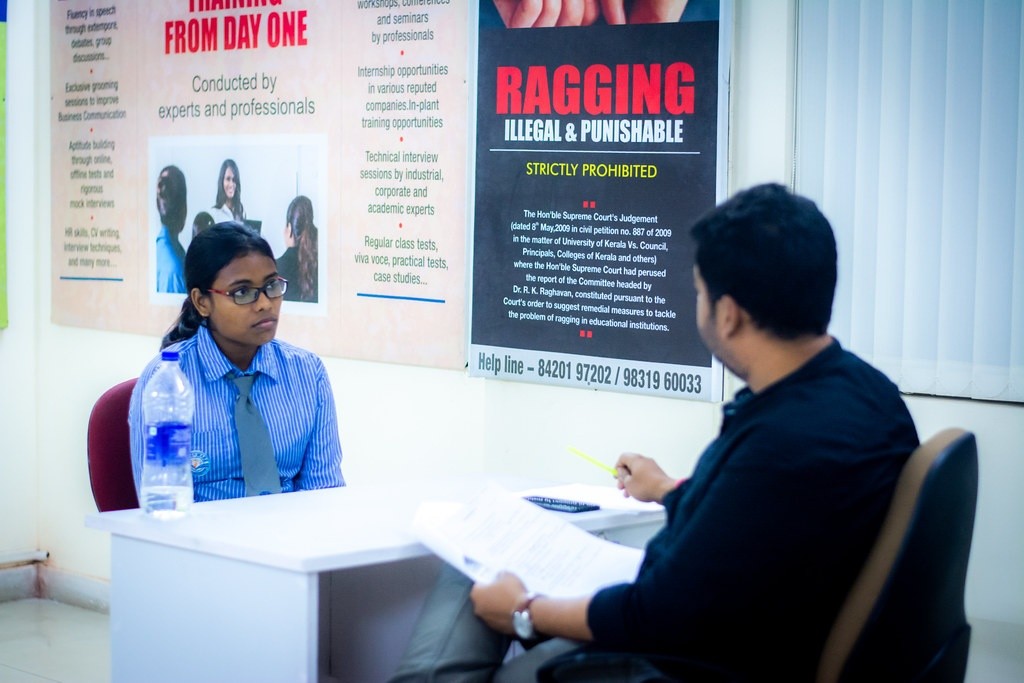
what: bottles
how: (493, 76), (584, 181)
(139, 351), (195, 522)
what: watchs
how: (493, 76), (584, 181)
(511, 593), (545, 640)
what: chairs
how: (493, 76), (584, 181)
(86, 378), (141, 512)
(815, 428), (979, 683)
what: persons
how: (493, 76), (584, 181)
(130, 221), (347, 507)
(156, 159), (319, 302)
(390, 184), (919, 683)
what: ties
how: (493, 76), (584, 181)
(224, 372), (282, 497)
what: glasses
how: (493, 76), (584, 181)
(208, 276), (287, 306)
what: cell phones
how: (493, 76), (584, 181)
(523, 497), (600, 514)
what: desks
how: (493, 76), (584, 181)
(85, 469), (667, 683)
